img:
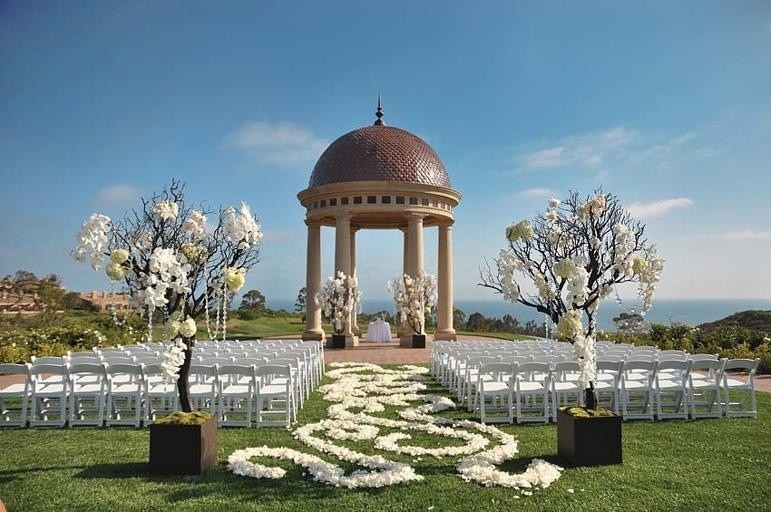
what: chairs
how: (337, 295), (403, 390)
(431, 340), (761, 426)
(0, 340), (324, 427)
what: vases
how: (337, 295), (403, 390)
(411, 335), (426, 348)
(150, 412), (218, 475)
(328, 334), (345, 348)
(556, 406), (624, 468)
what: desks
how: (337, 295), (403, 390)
(366, 321), (392, 341)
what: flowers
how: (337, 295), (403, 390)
(67, 178), (262, 413)
(385, 269), (439, 334)
(475, 183), (668, 410)
(312, 271), (362, 336)
